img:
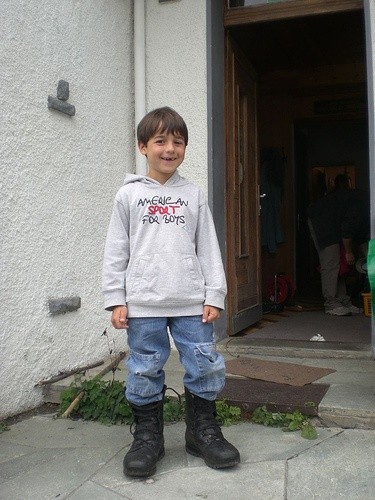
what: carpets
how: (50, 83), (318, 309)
(224, 356), (336, 388)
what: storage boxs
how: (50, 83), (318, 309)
(361, 289), (372, 320)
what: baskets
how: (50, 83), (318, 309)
(361, 294), (371, 316)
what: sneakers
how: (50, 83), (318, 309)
(348, 306), (363, 314)
(324, 306), (352, 316)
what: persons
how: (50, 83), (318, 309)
(305, 191), (370, 316)
(101, 106), (239, 476)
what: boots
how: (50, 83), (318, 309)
(123, 383), (182, 478)
(183, 386), (240, 468)
